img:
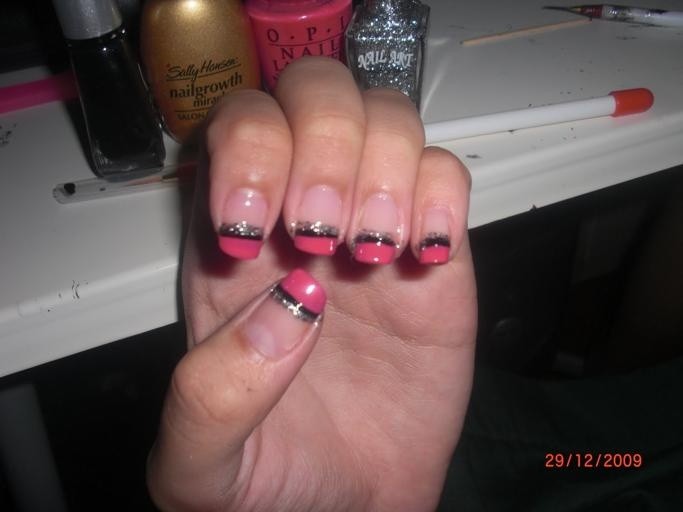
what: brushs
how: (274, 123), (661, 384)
(542, 4), (683, 27)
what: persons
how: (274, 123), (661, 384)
(145, 55), (479, 512)
